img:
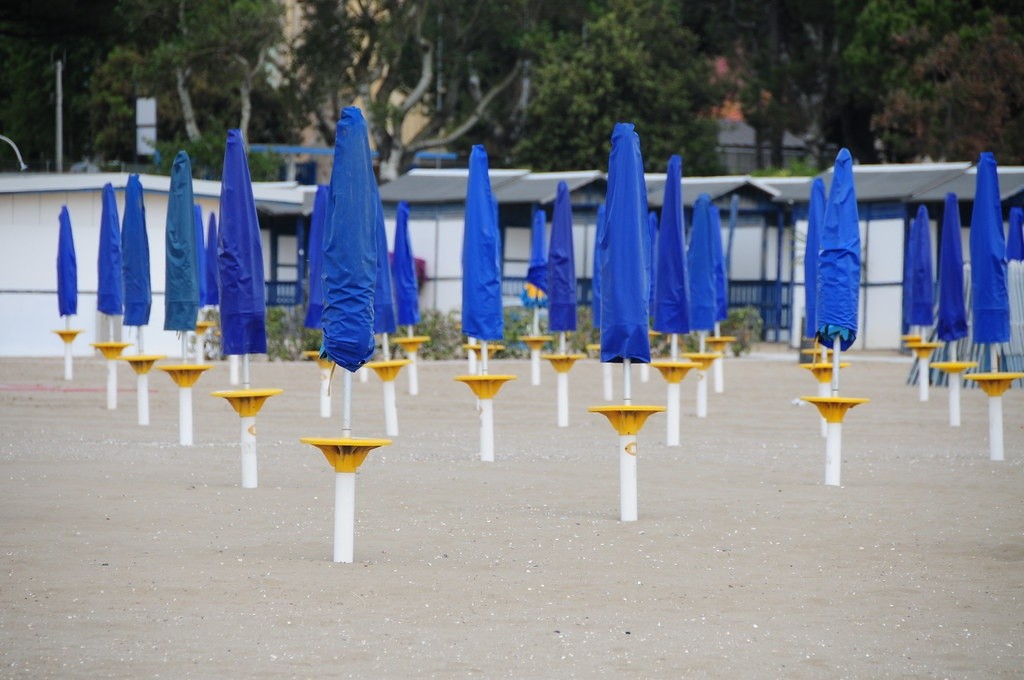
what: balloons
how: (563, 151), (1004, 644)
(588, 124), (666, 521)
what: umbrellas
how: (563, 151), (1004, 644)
(902, 151), (1024, 462)
(806, 148), (869, 486)
(195, 203), (220, 364)
(305, 186), (431, 437)
(453, 145), (611, 462)
(123, 173), (163, 426)
(210, 128), (284, 487)
(156, 150), (214, 446)
(640, 156), (735, 448)
(88, 183), (131, 410)
(300, 107), (391, 561)
(53, 206), (82, 381)
